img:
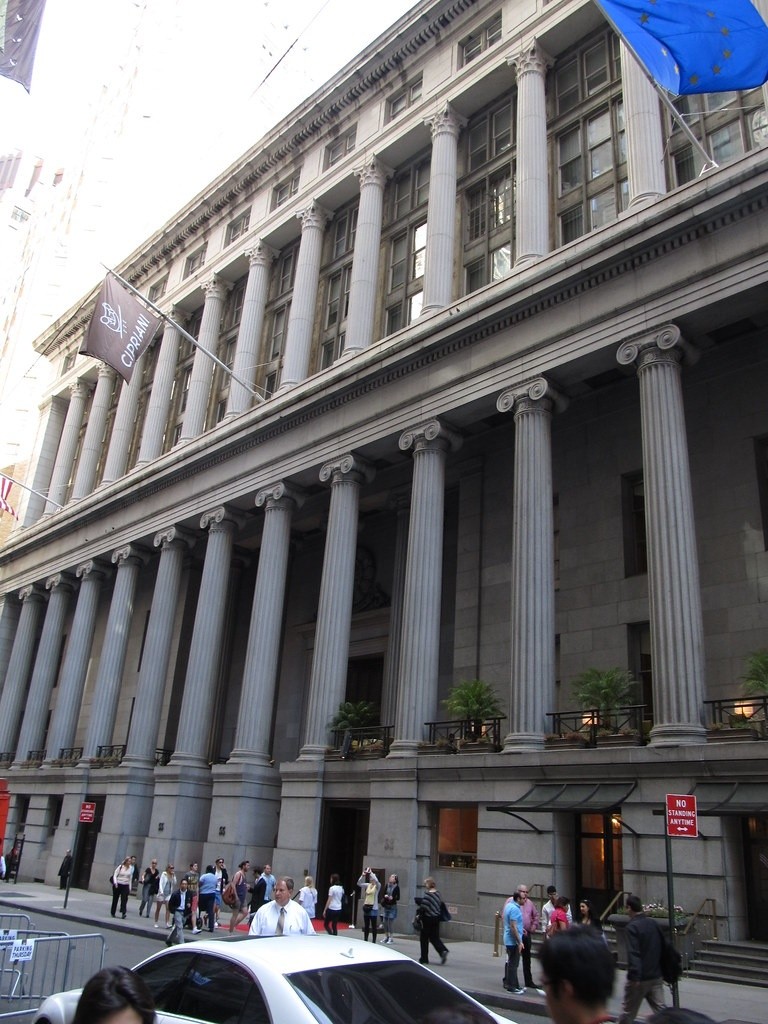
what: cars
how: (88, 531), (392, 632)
(31, 932), (519, 1024)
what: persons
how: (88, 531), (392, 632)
(357, 868), (381, 943)
(322, 874), (344, 935)
(57, 849), (73, 890)
(72, 965), (156, 1024)
(247, 876), (316, 935)
(380, 874), (400, 945)
(618, 896), (667, 1024)
(299, 876), (318, 919)
(415, 876), (450, 965)
(502, 884), (573, 995)
(111, 856), (277, 935)
(0, 848), (17, 883)
(165, 878), (193, 947)
(575, 900), (602, 930)
(535, 923), (620, 1024)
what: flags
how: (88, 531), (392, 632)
(77, 271), (163, 387)
(0, 476), (18, 520)
(595, 0), (768, 98)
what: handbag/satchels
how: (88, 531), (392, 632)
(381, 898), (390, 909)
(363, 904), (373, 913)
(222, 884), (236, 905)
(413, 920), (424, 931)
(440, 900), (452, 923)
(109, 876), (113, 884)
(546, 922), (559, 938)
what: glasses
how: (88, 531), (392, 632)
(519, 890), (528, 893)
(219, 862), (224, 863)
(170, 867), (174, 869)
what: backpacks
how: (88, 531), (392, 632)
(652, 920), (683, 983)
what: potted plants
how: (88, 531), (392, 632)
(545, 732), (587, 750)
(0, 761), (11, 771)
(51, 757), (78, 769)
(707, 722), (758, 742)
(89, 755), (119, 769)
(417, 737), (451, 754)
(357, 744), (387, 760)
(596, 727), (642, 747)
(323, 747), (349, 761)
(459, 737), (496, 753)
(20, 761), (42, 770)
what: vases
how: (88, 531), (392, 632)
(608, 914), (694, 970)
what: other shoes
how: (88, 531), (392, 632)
(154, 924), (159, 928)
(380, 937), (394, 944)
(441, 950), (449, 965)
(214, 921), (219, 929)
(122, 915), (126, 919)
(193, 929), (202, 935)
(165, 924), (172, 929)
(525, 982), (537, 989)
(139, 911), (142, 917)
(166, 941), (173, 947)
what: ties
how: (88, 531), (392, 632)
(274, 908), (285, 934)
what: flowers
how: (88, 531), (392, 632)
(617, 896), (686, 921)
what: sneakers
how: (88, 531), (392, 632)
(507, 986), (528, 995)
